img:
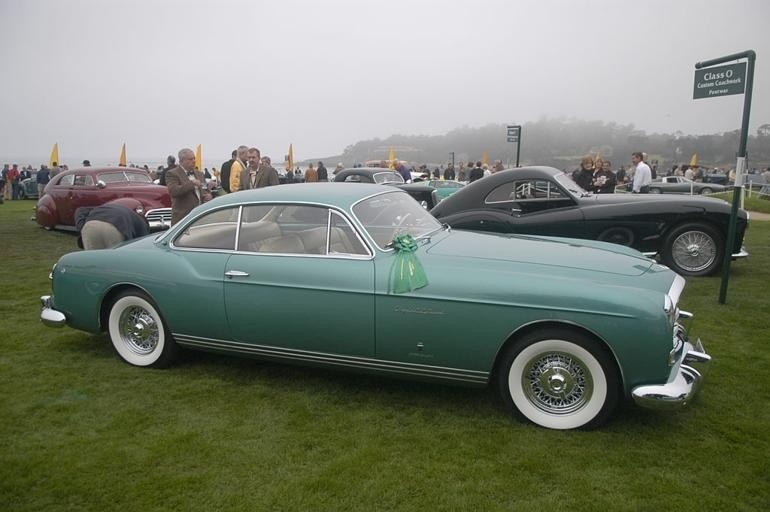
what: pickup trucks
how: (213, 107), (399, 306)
(649, 175), (727, 195)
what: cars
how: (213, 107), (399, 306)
(332, 166), (469, 208)
(18, 175), (53, 200)
(28, 165), (172, 235)
(38, 177), (713, 432)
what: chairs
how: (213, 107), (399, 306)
(259, 227), (356, 254)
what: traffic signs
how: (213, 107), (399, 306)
(507, 129), (519, 136)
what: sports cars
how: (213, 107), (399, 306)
(426, 163), (749, 277)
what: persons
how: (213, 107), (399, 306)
(572, 151), (657, 194)
(1, 159), (91, 201)
(380, 159), (506, 185)
(667, 163), (769, 185)
(75, 205), (150, 252)
(128, 145), (362, 230)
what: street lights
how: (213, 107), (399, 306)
(692, 61), (747, 100)
(449, 151), (454, 168)
(506, 138), (519, 143)
